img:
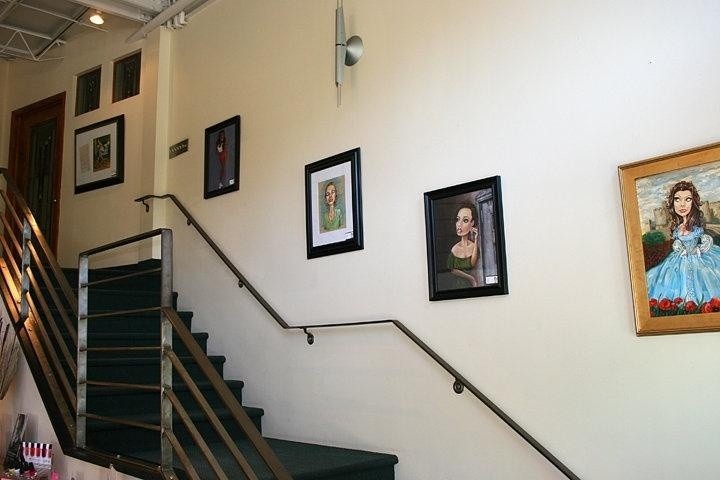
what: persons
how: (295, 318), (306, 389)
(646, 181), (720, 310)
(446, 201), (480, 289)
(319, 182), (343, 233)
(216, 129), (229, 187)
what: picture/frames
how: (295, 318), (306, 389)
(618, 141), (719, 337)
(75, 114), (125, 195)
(203, 115), (240, 199)
(423, 174), (510, 301)
(304, 148), (364, 260)
